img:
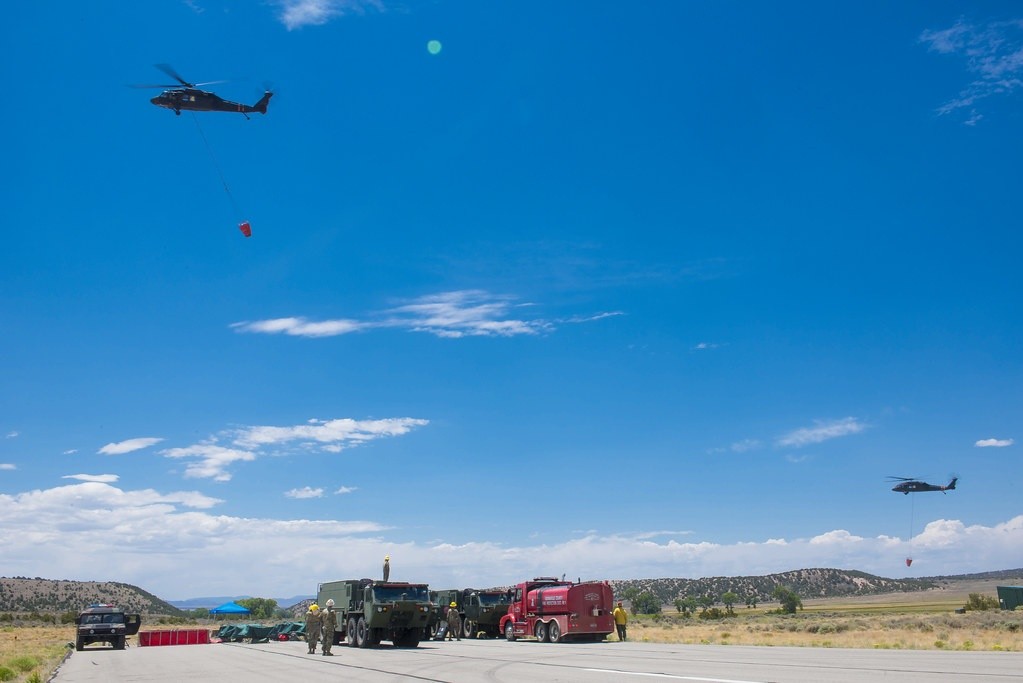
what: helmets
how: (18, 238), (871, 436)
(326, 599), (335, 607)
(385, 556), (389, 560)
(309, 604), (319, 611)
(450, 602), (457, 606)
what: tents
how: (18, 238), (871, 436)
(207, 602), (251, 625)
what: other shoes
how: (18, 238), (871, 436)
(307, 651), (315, 654)
(323, 651), (333, 656)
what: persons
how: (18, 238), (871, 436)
(447, 602), (461, 641)
(306, 604), (321, 654)
(319, 599), (338, 657)
(382, 556), (390, 582)
(613, 602), (627, 642)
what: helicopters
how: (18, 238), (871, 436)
(883, 476), (959, 496)
(125, 63), (275, 120)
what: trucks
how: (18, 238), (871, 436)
(499, 573), (615, 643)
(314, 578), (435, 649)
(421, 587), (518, 642)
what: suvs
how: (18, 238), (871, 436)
(74, 603), (142, 651)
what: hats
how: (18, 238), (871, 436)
(617, 601), (623, 607)
